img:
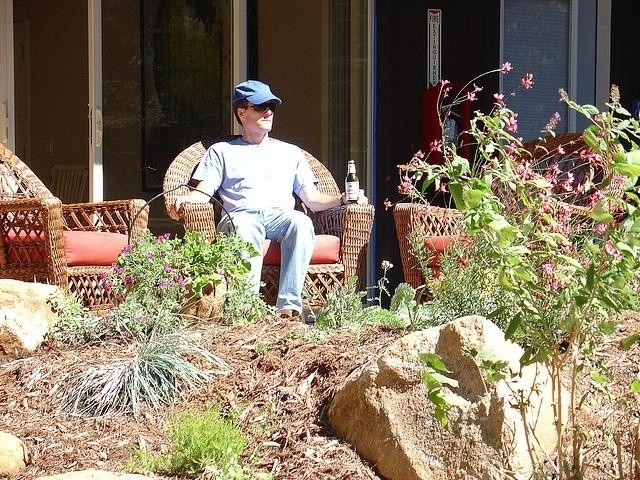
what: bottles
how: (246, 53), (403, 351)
(345, 158), (360, 204)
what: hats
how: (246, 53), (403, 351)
(232, 80), (282, 105)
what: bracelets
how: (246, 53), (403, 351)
(339, 196), (346, 206)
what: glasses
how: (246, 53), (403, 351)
(244, 102), (276, 112)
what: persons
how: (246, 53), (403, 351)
(167, 79), (370, 324)
